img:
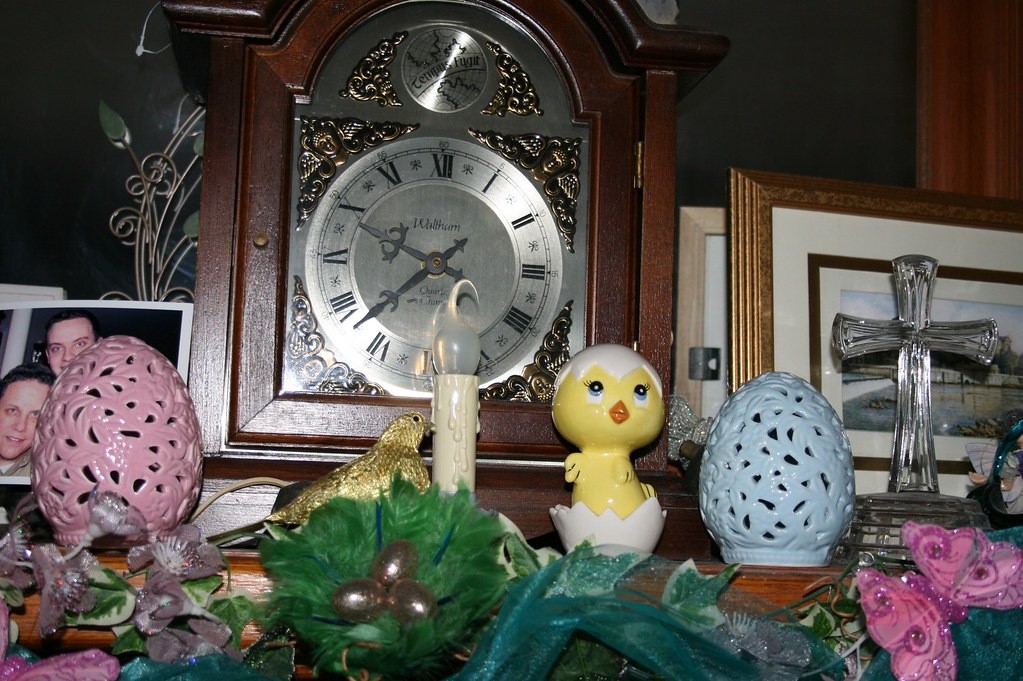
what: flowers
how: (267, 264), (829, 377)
(0, 489), (233, 680)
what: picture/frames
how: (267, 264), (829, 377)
(675, 208), (729, 416)
(731, 168), (1023, 524)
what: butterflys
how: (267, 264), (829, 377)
(850, 515), (1022, 681)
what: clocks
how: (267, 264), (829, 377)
(162, 0), (732, 482)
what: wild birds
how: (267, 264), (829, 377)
(265, 412), (437, 525)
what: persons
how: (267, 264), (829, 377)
(0, 313), (101, 477)
